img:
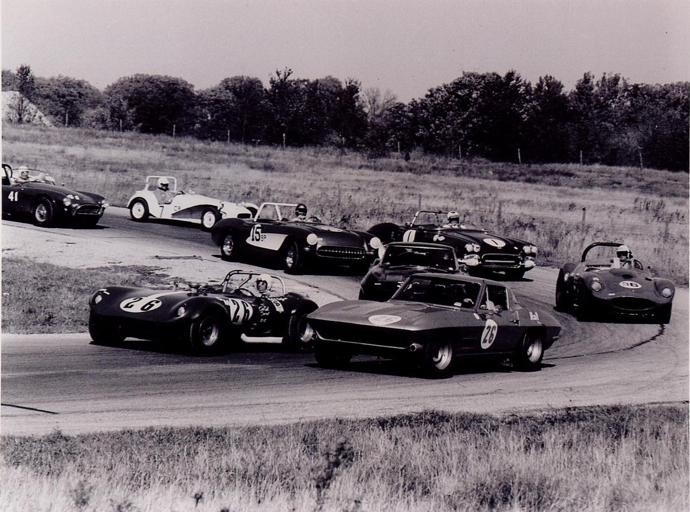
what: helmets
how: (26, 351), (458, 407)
(256, 274), (272, 290)
(447, 211), (459, 222)
(617, 246), (630, 259)
(295, 204), (307, 216)
(18, 166), (29, 177)
(158, 178), (169, 188)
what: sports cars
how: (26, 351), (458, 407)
(89, 270), (319, 357)
(555, 242), (675, 324)
(127, 176), (258, 233)
(1, 163), (108, 227)
(211, 202), (384, 275)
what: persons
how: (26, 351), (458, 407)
(16, 165), (36, 185)
(290, 203), (312, 223)
(611, 245), (634, 269)
(156, 177), (171, 204)
(254, 274), (280, 298)
(442, 211), (463, 229)
